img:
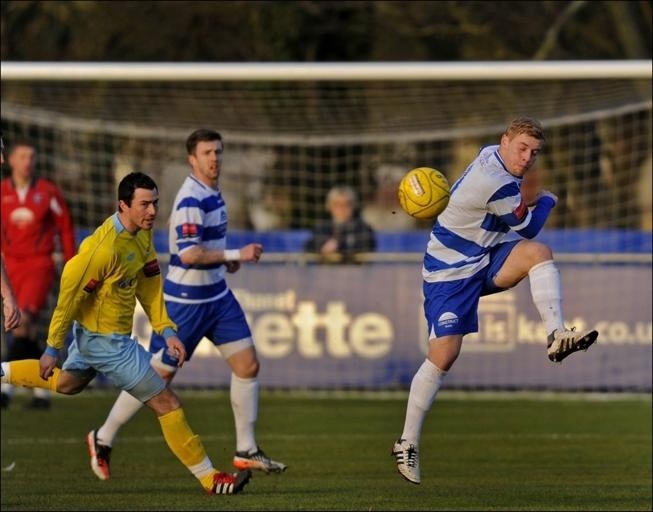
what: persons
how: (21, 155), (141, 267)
(2, 2), (652, 270)
(83, 130), (290, 484)
(0, 254), (22, 332)
(0, 140), (77, 412)
(389, 114), (599, 486)
(0, 170), (252, 498)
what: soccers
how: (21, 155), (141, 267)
(397, 167), (450, 220)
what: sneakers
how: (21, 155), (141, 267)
(232, 446), (286, 471)
(547, 327), (598, 362)
(208, 470), (250, 494)
(392, 439), (420, 484)
(87, 429), (111, 480)
